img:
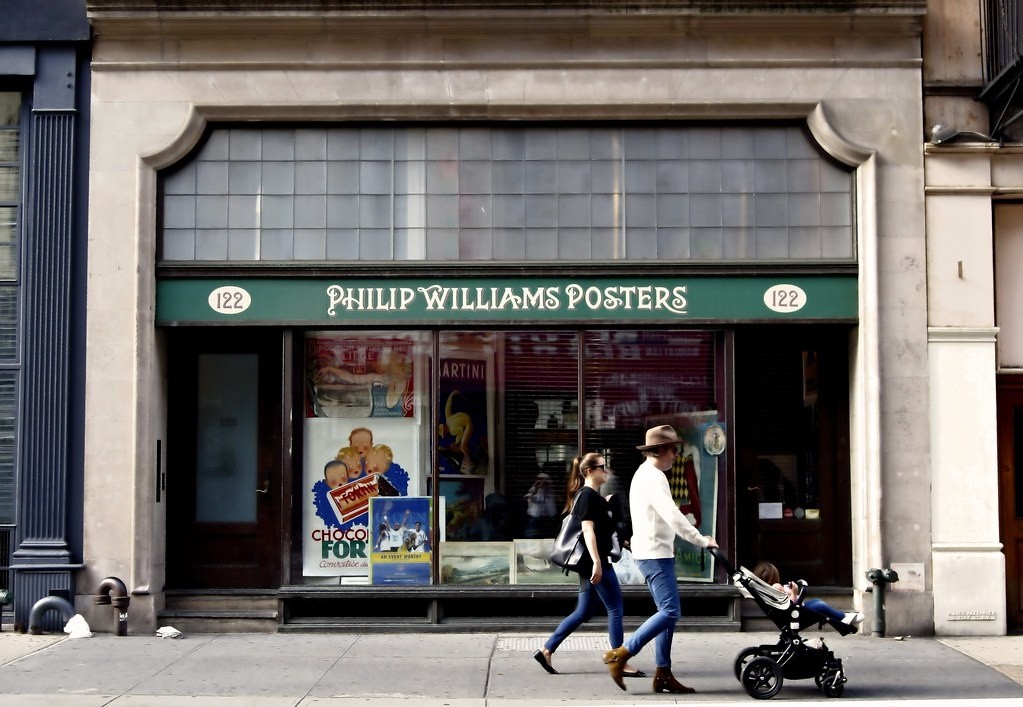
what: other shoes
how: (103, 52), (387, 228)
(622, 670), (646, 677)
(533, 650), (558, 674)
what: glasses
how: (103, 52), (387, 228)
(667, 445), (679, 456)
(589, 464), (606, 472)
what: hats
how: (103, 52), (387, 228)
(636, 425), (683, 449)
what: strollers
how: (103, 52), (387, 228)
(705, 542), (858, 699)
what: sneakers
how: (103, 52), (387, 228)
(841, 612), (866, 626)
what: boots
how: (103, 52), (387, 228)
(602, 646), (632, 692)
(652, 667), (695, 693)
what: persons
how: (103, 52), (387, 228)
(753, 561), (864, 627)
(534, 451), (647, 680)
(603, 424), (720, 694)
(524, 472), (563, 539)
(375, 506), (430, 553)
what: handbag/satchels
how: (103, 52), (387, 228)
(547, 489), (593, 576)
